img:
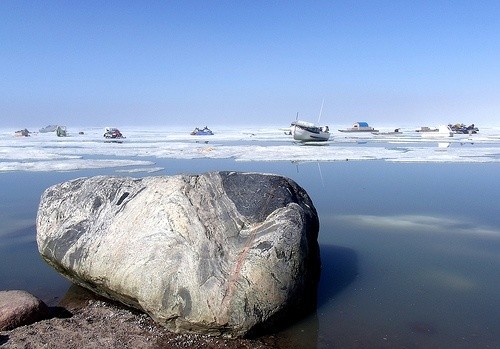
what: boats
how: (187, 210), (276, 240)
(193, 126), (214, 137)
(103, 129), (121, 139)
(290, 120), (333, 143)
(13, 126), (85, 137)
(339, 122), (379, 133)
(413, 124), (487, 142)
(371, 130), (404, 137)
